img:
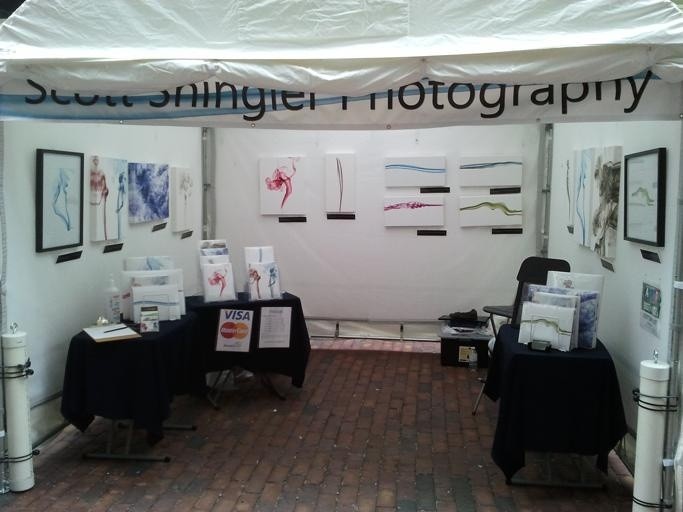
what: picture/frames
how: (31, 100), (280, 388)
(36, 148), (85, 253)
(623, 147), (667, 247)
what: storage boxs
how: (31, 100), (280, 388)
(440, 328), (492, 368)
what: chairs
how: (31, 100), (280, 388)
(485, 257), (571, 340)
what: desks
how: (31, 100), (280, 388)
(58, 292), (312, 462)
(485, 322), (628, 492)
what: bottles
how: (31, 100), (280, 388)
(468, 347), (477, 373)
